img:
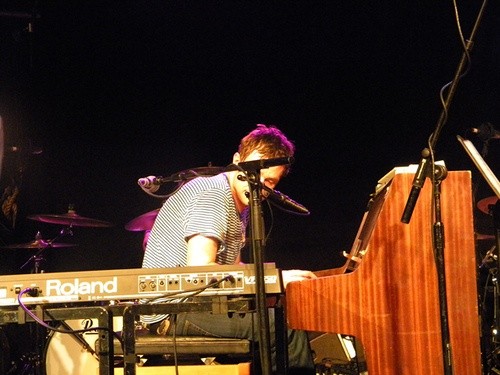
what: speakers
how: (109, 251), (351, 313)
(311, 332), (357, 375)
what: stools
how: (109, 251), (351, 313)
(96, 330), (250, 375)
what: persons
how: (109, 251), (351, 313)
(477, 138), (500, 218)
(141, 124), (316, 375)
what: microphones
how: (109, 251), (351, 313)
(137, 176), (161, 192)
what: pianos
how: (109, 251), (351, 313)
(281, 165), (487, 374)
(0, 263), (282, 375)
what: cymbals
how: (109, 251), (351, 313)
(0, 242), (83, 250)
(24, 214), (115, 228)
